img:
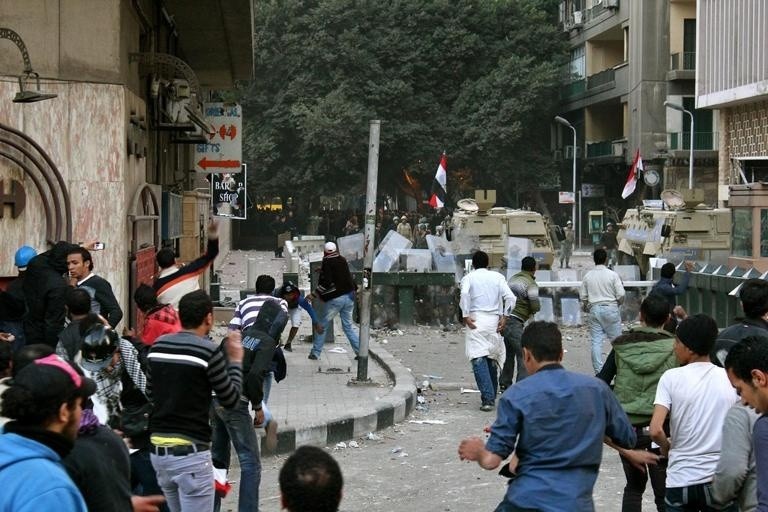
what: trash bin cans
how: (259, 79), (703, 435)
(283, 273), (298, 287)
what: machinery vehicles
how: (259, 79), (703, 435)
(616, 189), (733, 297)
(447, 189), (556, 275)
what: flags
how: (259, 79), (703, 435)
(620, 147), (644, 200)
(427, 149), (447, 214)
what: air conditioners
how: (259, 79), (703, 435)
(572, 11), (582, 24)
(565, 145), (574, 160)
(603, 0), (619, 8)
(554, 151), (562, 161)
(611, 144), (623, 155)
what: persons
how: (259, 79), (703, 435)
(271, 281), (325, 352)
(499, 256), (541, 394)
(229, 275), (289, 453)
(212, 299), (289, 512)
(458, 251), (517, 412)
(600, 222), (618, 267)
(579, 248), (628, 385)
(304, 241), (359, 361)
(1, 218), (244, 511)
(596, 259), (766, 512)
(560, 220), (574, 268)
(272, 210), (300, 258)
(278, 444), (343, 512)
(457, 320), (638, 511)
(344, 207), (452, 249)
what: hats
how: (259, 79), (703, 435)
(323, 242), (339, 255)
(392, 215), (409, 222)
(13, 244), (37, 269)
(674, 313), (719, 356)
(5, 352), (97, 411)
(566, 220), (572, 225)
(282, 281), (295, 293)
(606, 222), (613, 226)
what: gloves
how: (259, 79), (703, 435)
(313, 322), (325, 334)
(303, 294), (316, 304)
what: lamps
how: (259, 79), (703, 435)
(0, 28), (57, 103)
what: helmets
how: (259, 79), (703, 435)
(79, 321), (118, 375)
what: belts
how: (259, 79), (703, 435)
(146, 442), (209, 458)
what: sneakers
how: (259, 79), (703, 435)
(479, 402), (495, 412)
(307, 352), (318, 359)
(283, 343), (291, 351)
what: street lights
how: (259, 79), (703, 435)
(552, 115), (577, 250)
(660, 101), (695, 191)
